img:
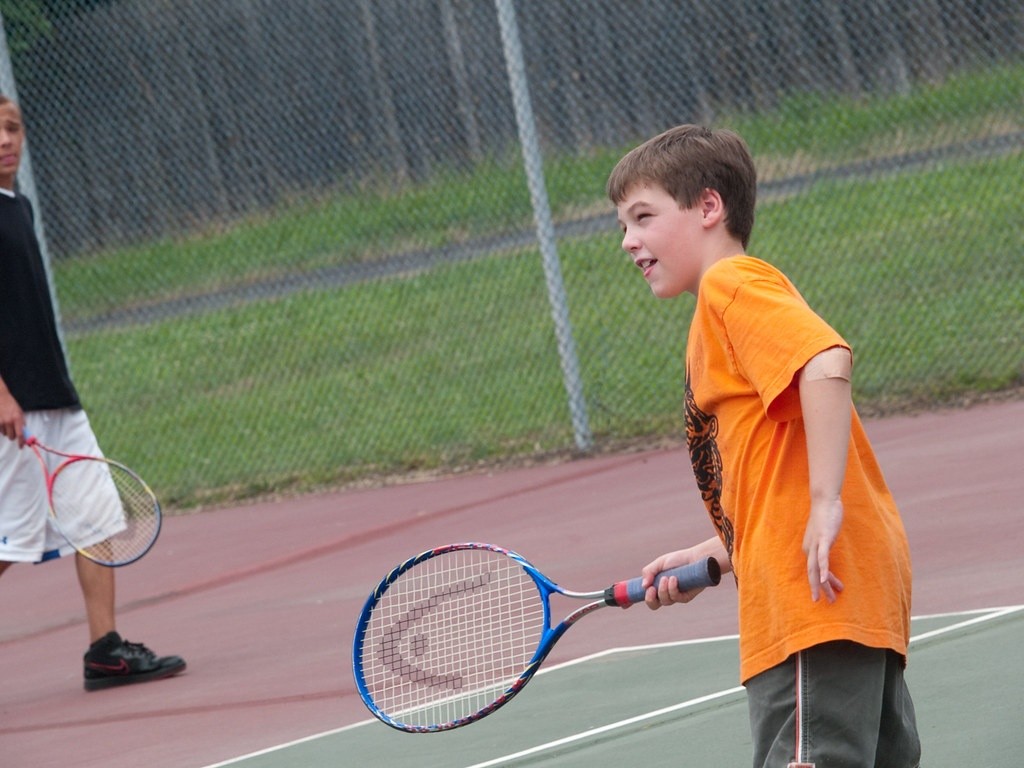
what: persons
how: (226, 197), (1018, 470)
(608, 124), (922, 768)
(0, 97), (186, 690)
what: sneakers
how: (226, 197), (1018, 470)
(83, 641), (187, 692)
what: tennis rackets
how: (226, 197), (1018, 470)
(20, 429), (162, 568)
(352, 542), (721, 734)
